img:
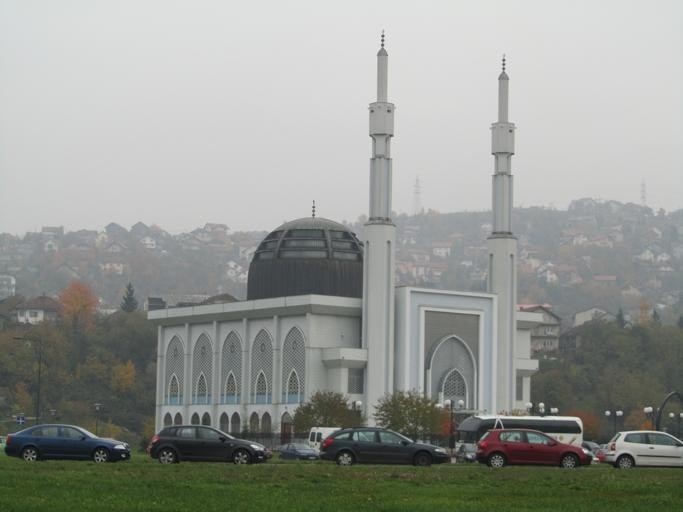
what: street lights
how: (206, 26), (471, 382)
(604, 408), (623, 436)
(642, 407), (660, 430)
(94, 402), (103, 436)
(668, 411), (683, 439)
(49, 408), (55, 422)
(549, 407), (558, 416)
(524, 400), (548, 417)
(355, 399), (362, 424)
(444, 399), (464, 449)
(12, 336), (41, 423)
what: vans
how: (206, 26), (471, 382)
(307, 426), (369, 458)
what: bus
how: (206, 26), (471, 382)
(453, 415), (583, 464)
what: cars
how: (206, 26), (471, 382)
(4, 422), (130, 462)
(582, 439), (608, 462)
(473, 428), (592, 468)
(320, 427), (451, 467)
(598, 431), (683, 469)
(145, 424), (273, 464)
(277, 443), (319, 462)
